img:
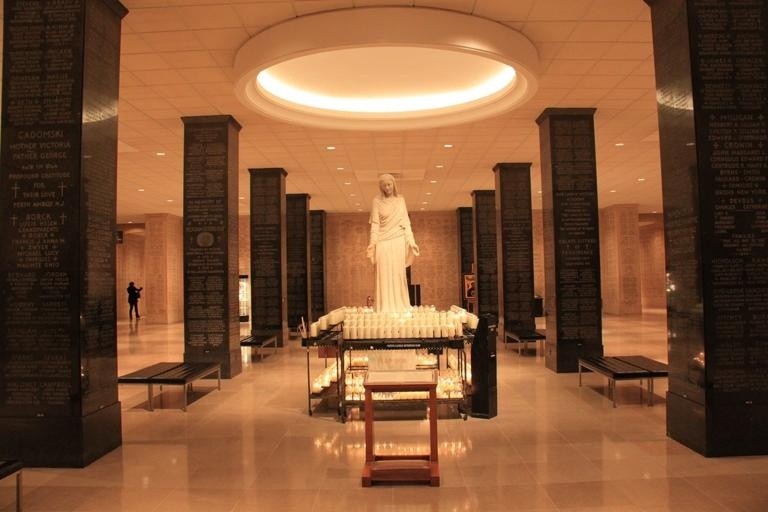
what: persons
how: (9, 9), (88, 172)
(367, 173), (419, 311)
(127, 282), (143, 320)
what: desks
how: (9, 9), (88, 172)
(360, 368), (442, 488)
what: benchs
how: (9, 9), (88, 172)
(240, 335), (278, 360)
(575, 352), (669, 409)
(504, 328), (545, 358)
(116, 360), (224, 412)
